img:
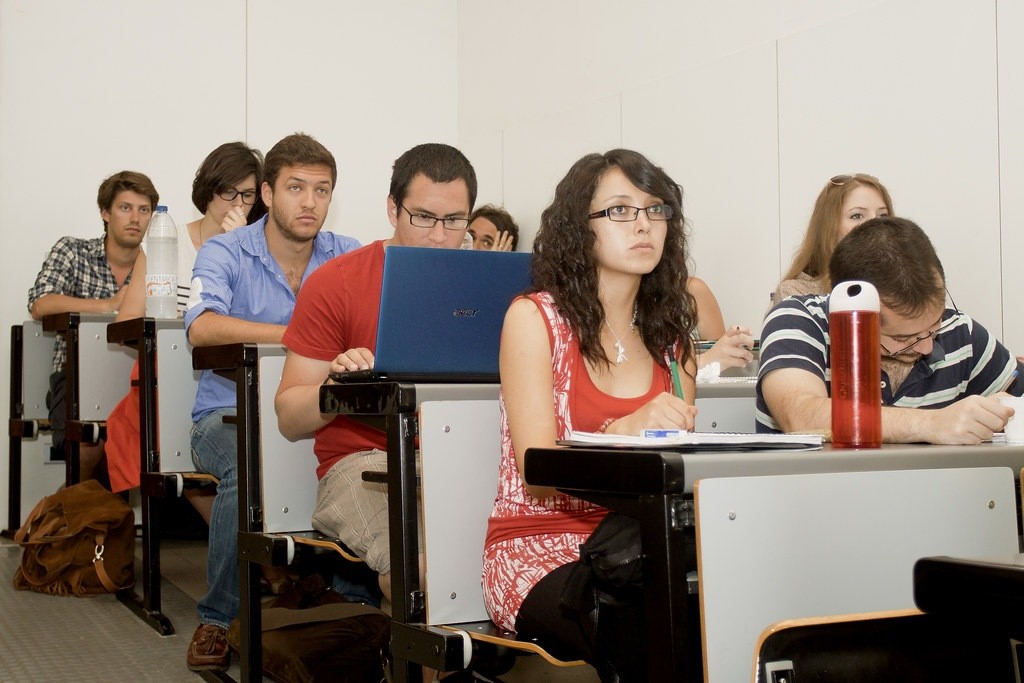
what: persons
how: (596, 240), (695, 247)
(185, 130), (384, 671)
(686, 277), (754, 373)
(467, 204), (519, 251)
(275, 142), (477, 683)
(106, 141), (302, 594)
(773, 172), (896, 304)
(484, 148), (703, 683)
(27, 171), (159, 481)
(754, 216), (1024, 445)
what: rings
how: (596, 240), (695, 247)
(500, 246), (503, 248)
(240, 213), (244, 216)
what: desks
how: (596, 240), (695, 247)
(189, 342), (324, 535)
(42, 310), (140, 420)
(522, 441), (1024, 683)
(913, 553), (1024, 683)
(319, 374), (755, 619)
(106, 317), (206, 476)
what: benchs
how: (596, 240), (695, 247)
(0, 309), (1024, 683)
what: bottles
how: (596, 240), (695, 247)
(145, 206), (178, 319)
(829, 280), (883, 449)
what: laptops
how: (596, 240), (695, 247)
(328, 246), (537, 382)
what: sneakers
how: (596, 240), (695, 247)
(186, 619), (232, 671)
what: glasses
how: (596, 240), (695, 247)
(587, 204), (674, 222)
(392, 198), (470, 230)
(880, 284), (959, 359)
(218, 184), (260, 205)
(826, 173), (879, 199)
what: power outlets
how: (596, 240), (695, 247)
(43, 443), (66, 464)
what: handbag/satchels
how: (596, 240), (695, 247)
(223, 575), (393, 683)
(559, 512), (652, 683)
(12, 478), (136, 598)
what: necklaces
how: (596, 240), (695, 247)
(604, 317), (631, 363)
(200, 217), (204, 247)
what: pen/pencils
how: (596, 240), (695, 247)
(736, 326), (740, 334)
(667, 346), (684, 400)
(1000, 371), (1018, 392)
